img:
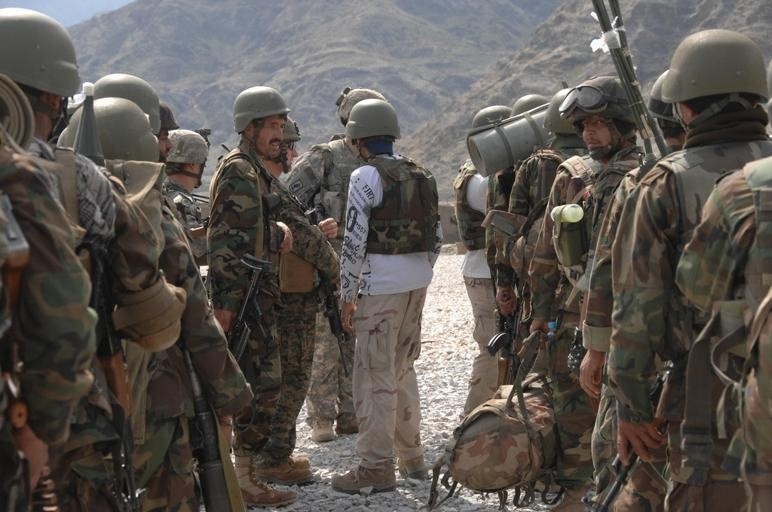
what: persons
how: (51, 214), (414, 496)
(255, 118), (340, 484)
(206, 86), (299, 509)
(454, 28), (772, 512)
(284, 88), (385, 442)
(330, 98), (441, 494)
(2, 7), (251, 512)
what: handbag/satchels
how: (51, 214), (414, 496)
(448, 371), (558, 490)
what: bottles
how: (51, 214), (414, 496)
(550, 204), (584, 223)
(546, 321), (558, 337)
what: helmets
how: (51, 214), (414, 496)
(340, 88), (400, 139)
(1, 8), (207, 164)
(472, 28), (768, 141)
(234, 86), (301, 141)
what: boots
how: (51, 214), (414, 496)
(235, 412), (427, 507)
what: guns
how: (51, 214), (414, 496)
(226, 253), (271, 362)
(177, 337), (245, 511)
(487, 293), (522, 388)
(95, 295), (146, 512)
(0, 189), (58, 512)
(580, 362), (669, 511)
(304, 207), (350, 378)
